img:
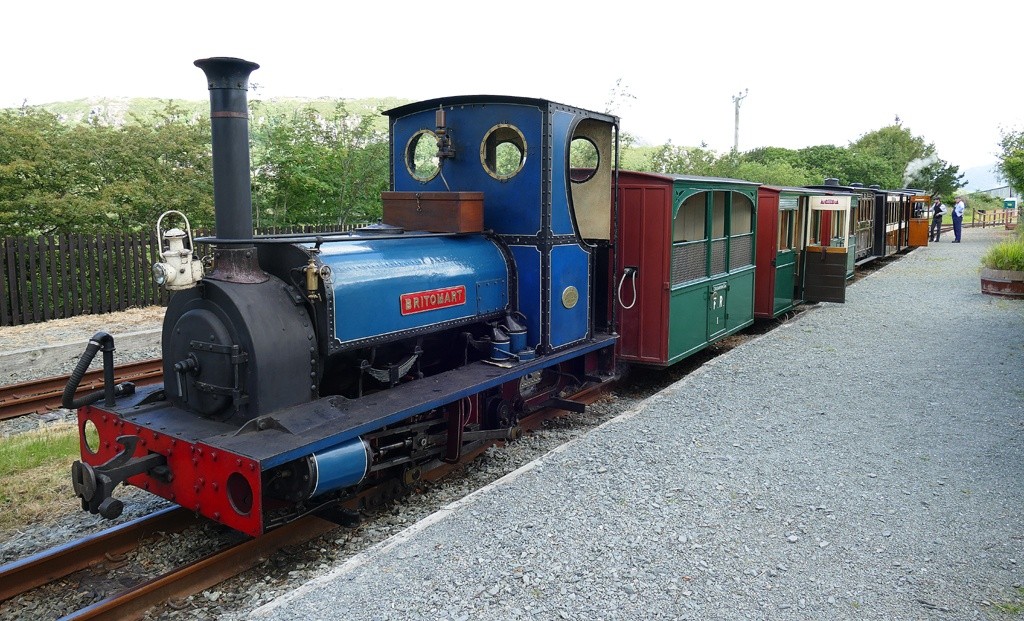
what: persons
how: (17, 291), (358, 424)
(920, 197), (947, 241)
(952, 196), (964, 243)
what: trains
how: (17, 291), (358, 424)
(60, 56), (931, 540)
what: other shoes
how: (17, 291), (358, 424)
(952, 240), (960, 243)
(935, 239), (939, 242)
(929, 239), (933, 242)
